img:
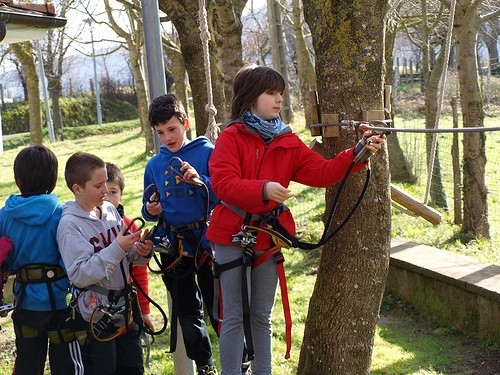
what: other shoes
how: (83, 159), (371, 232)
(196, 364), (218, 375)
(242, 360), (253, 375)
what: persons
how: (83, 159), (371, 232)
(104, 163), (155, 332)
(142, 94), (252, 375)
(204, 65), (386, 375)
(57, 153), (154, 375)
(1, 146), (87, 375)
